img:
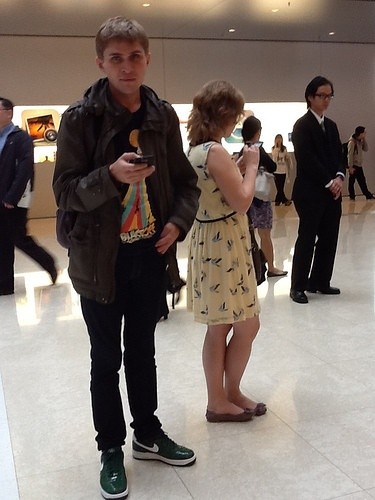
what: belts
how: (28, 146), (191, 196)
(119, 223), (157, 244)
(193, 210), (237, 223)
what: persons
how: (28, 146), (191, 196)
(185, 80), (267, 423)
(53, 15), (200, 500)
(271, 134), (292, 206)
(0, 98), (56, 296)
(347, 126), (375, 200)
(238, 115), (288, 276)
(289, 76), (346, 303)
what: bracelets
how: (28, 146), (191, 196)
(336, 174), (344, 181)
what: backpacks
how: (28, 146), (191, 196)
(341, 140), (358, 169)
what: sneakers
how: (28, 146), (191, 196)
(132, 428), (196, 466)
(99, 449), (129, 499)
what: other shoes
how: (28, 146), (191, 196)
(205, 405), (255, 422)
(275, 201), (280, 206)
(0, 290), (15, 296)
(49, 262), (59, 284)
(244, 403), (267, 415)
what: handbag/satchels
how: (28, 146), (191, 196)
(255, 168), (278, 202)
(250, 244), (267, 286)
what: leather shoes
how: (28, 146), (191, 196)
(285, 201), (292, 206)
(308, 287), (340, 294)
(289, 288), (308, 303)
(267, 270), (288, 277)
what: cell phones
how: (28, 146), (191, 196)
(253, 141), (264, 148)
(132, 153), (156, 168)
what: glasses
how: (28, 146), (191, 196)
(315, 92), (334, 100)
